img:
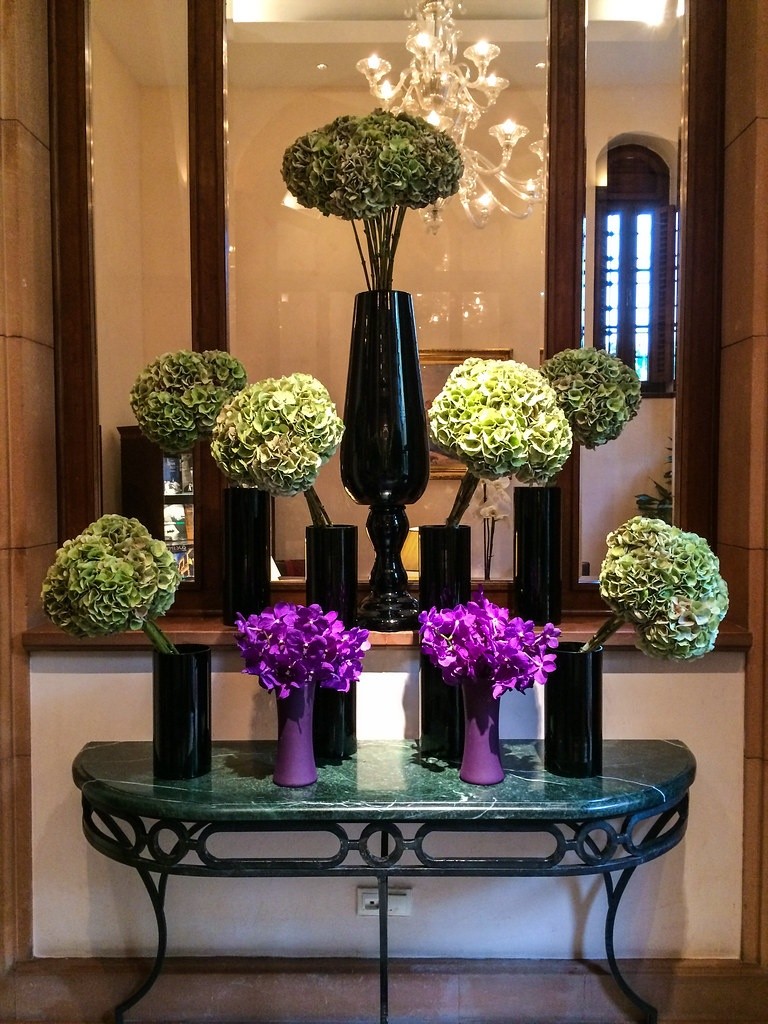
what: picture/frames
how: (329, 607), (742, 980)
(419, 348), (513, 480)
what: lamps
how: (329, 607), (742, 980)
(356, 0), (544, 235)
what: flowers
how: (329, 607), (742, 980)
(39, 514), (182, 653)
(540, 348), (642, 450)
(130, 349), (247, 453)
(418, 584), (562, 700)
(233, 601), (371, 699)
(427, 356), (573, 525)
(210, 372), (346, 527)
(474, 477), (515, 582)
(579, 515), (729, 662)
(280, 109), (462, 291)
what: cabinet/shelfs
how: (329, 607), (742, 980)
(116, 426), (222, 617)
(72, 740), (696, 1024)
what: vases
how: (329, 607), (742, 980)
(339, 290), (430, 631)
(420, 525), (471, 759)
(222, 485), (270, 626)
(460, 679), (504, 785)
(273, 680), (317, 787)
(514, 487), (561, 626)
(306, 524), (358, 757)
(545, 642), (603, 779)
(153, 644), (212, 780)
